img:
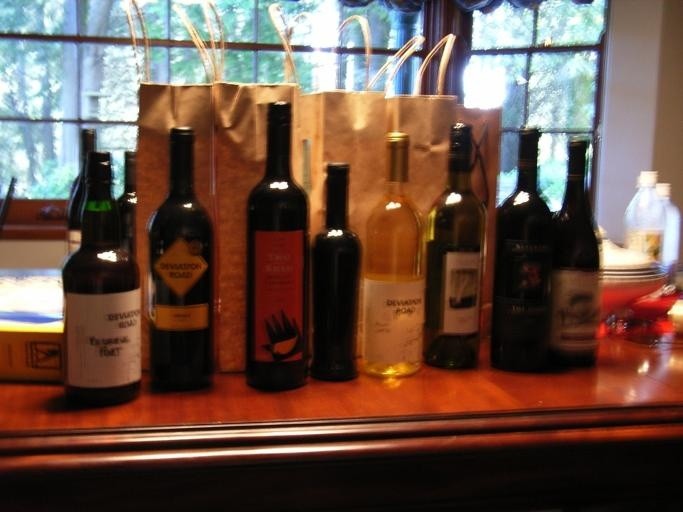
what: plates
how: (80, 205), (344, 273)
(599, 258), (668, 293)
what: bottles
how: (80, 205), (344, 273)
(358, 132), (425, 380)
(423, 122), (487, 371)
(642, 184), (679, 264)
(245, 100), (309, 393)
(145, 127), (217, 393)
(309, 162), (362, 382)
(550, 137), (600, 367)
(60, 129), (142, 407)
(491, 127), (551, 373)
(623, 170), (657, 253)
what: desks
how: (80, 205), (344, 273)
(2, 275), (682, 512)
